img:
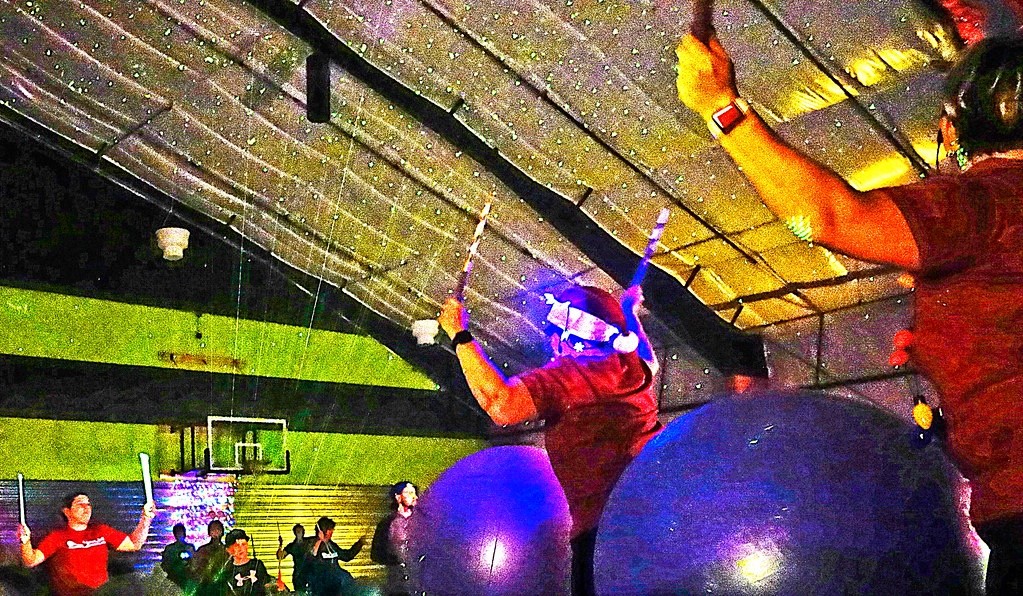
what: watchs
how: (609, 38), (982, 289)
(450, 329), (474, 353)
(705, 99), (753, 140)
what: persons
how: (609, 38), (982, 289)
(1, 449), (421, 595)
(672, 28), (1022, 595)
(437, 283), (664, 594)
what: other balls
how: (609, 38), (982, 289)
(594, 387), (991, 596)
(405, 445), (573, 596)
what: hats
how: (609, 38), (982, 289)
(390, 480), (412, 508)
(225, 529), (250, 548)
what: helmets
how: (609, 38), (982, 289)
(545, 286), (626, 345)
(942, 30), (1023, 155)
